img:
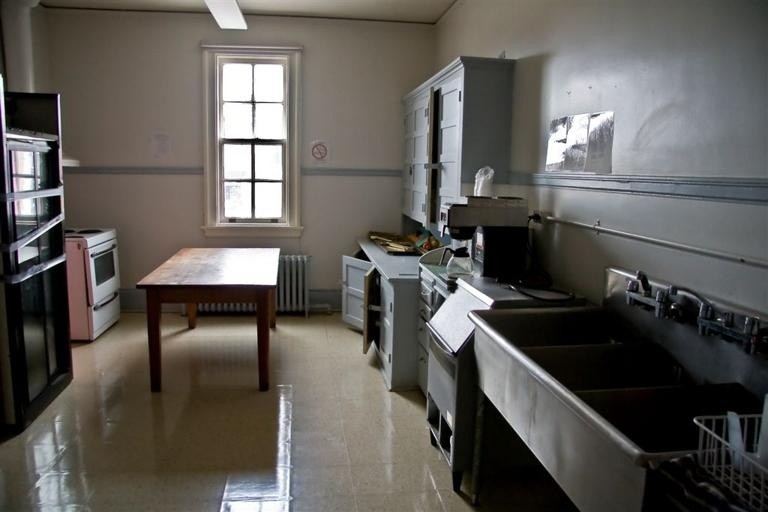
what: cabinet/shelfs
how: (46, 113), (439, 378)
(417, 266), (435, 396)
(403, 57), (512, 235)
(356, 247), (419, 389)
(0, 75), (74, 430)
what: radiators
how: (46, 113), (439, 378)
(179, 253), (313, 318)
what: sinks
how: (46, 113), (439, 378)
(517, 347), (739, 390)
(466, 307), (655, 346)
(569, 384), (767, 459)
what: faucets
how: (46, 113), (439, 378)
(634, 268), (653, 299)
(668, 285), (711, 319)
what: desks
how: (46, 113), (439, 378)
(136, 245), (281, 391)
